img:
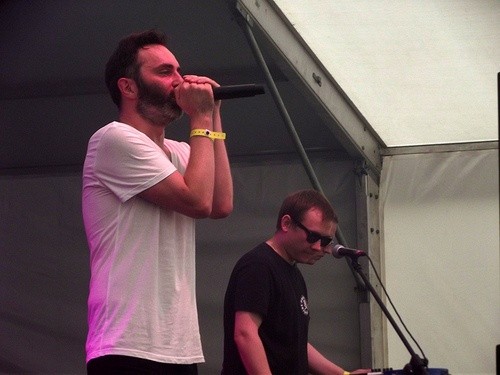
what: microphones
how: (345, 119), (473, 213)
(212, 83), (264, 102)
(332, 243), (366, 258)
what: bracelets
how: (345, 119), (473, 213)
(190, 129), (226, 142)
(344, 371), (350, 375)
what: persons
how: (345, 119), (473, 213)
(224, 191), (371, 375)
(82, 29), (232, 375)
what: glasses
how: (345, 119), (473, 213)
(295, 221), (331, 247)
